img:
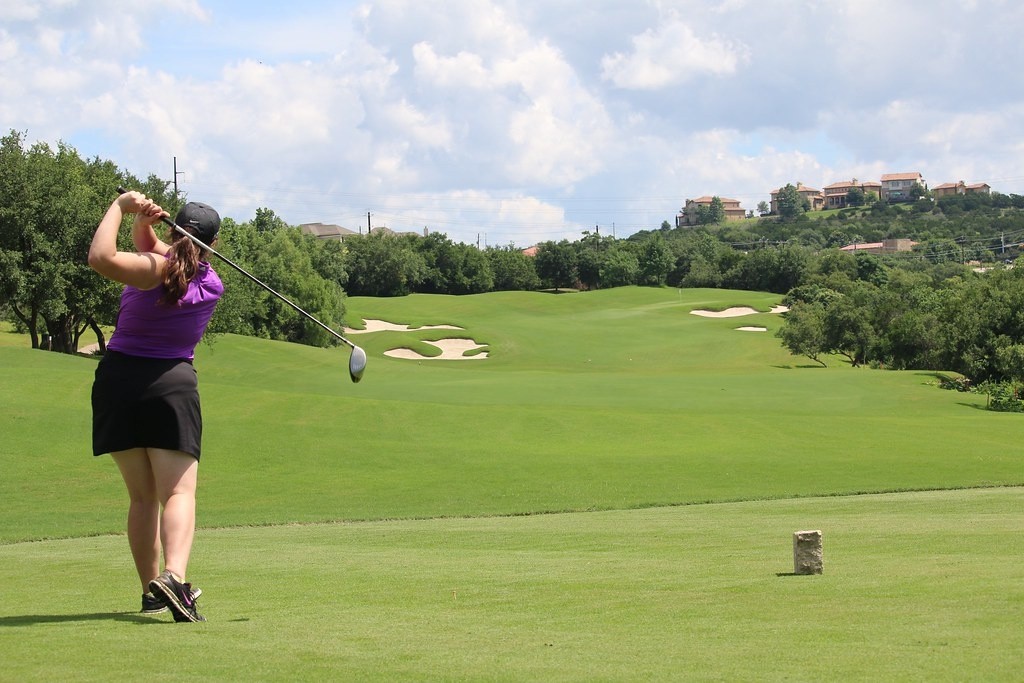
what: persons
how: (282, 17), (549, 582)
(88, 191), (223, 622)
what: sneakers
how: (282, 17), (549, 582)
(141, 593), (168, 613)
(149, 569), (207, 623)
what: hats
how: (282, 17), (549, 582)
(175, 203), (221, 248)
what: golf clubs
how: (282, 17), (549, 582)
(115, 183), (368, 385)
